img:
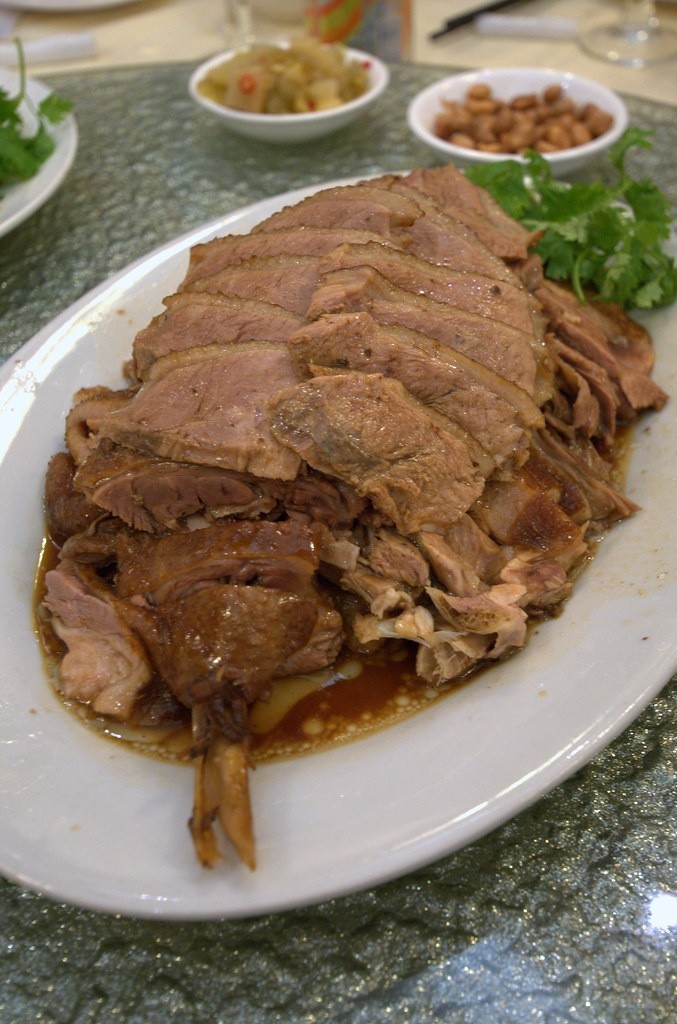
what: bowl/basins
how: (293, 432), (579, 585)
(187, 43), (389, 145)
(403, 65), (631, 178)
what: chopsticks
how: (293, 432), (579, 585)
(430, 0), (533, 40)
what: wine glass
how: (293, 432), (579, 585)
(575, 0), (677, 66)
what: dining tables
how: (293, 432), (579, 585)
(0, 55), (677, 1024)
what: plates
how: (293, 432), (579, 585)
(2, 174), (676, 918)
(0, 64), (78, 243)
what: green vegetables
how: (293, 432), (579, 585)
(463, 126), (677, 311)
(0, 36), (78, 198)
